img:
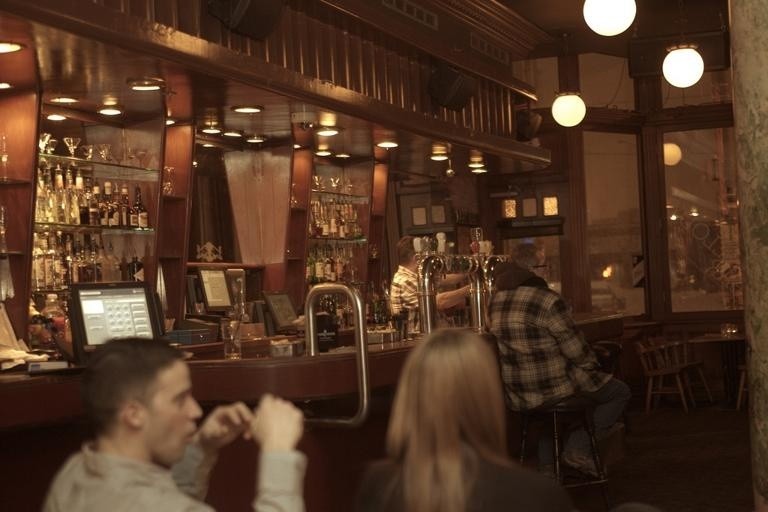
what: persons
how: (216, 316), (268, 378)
(38, 336), (310, 511)
(359, 326), (575, 510)
(388, 235), (470, 333)
(482, 242), (633, 479)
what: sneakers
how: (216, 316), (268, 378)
(562, 447), (607, 480)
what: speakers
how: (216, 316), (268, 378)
(207, 0), (283, 41)
(517, 109), (542, 141)
(628, 30), (730, 78)
(428, 68), (477, 112)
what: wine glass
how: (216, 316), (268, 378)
(312, 176), (325, 191)
(38, 132), (147, 167)
(330, 176), (341, 192)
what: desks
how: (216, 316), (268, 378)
(688, 332), (745, 406)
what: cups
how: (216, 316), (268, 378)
(219, 316), (243, 343)
(224, 342), (242, 360)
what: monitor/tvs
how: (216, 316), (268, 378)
(192, 268), (234, 312)
(66, 283), (166, 357)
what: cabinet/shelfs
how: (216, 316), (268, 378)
(224, 151), (390, 309)
(0, 95), (197, 349)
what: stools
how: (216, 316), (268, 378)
(735, 363), (749, 415)
(514, 404), (613, 512)
(589, 338), (630, 436)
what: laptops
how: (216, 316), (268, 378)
(262, 290), (326, 334)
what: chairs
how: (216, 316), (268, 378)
(635, 339), (694, 413)
(652, 332), (714, 406)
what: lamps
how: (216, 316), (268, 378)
(659, 0), (707, 90)
(580, 0), (639, 36)
(547, 32), (588, 130)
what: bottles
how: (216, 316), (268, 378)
(306, 283), (361, 327)
(36, 156), (157, 228)
(308, 197), (362, 240)
(366, 280), (390, 323)
(306, 243), (358, 282)
(31, 230), (145, 289)
